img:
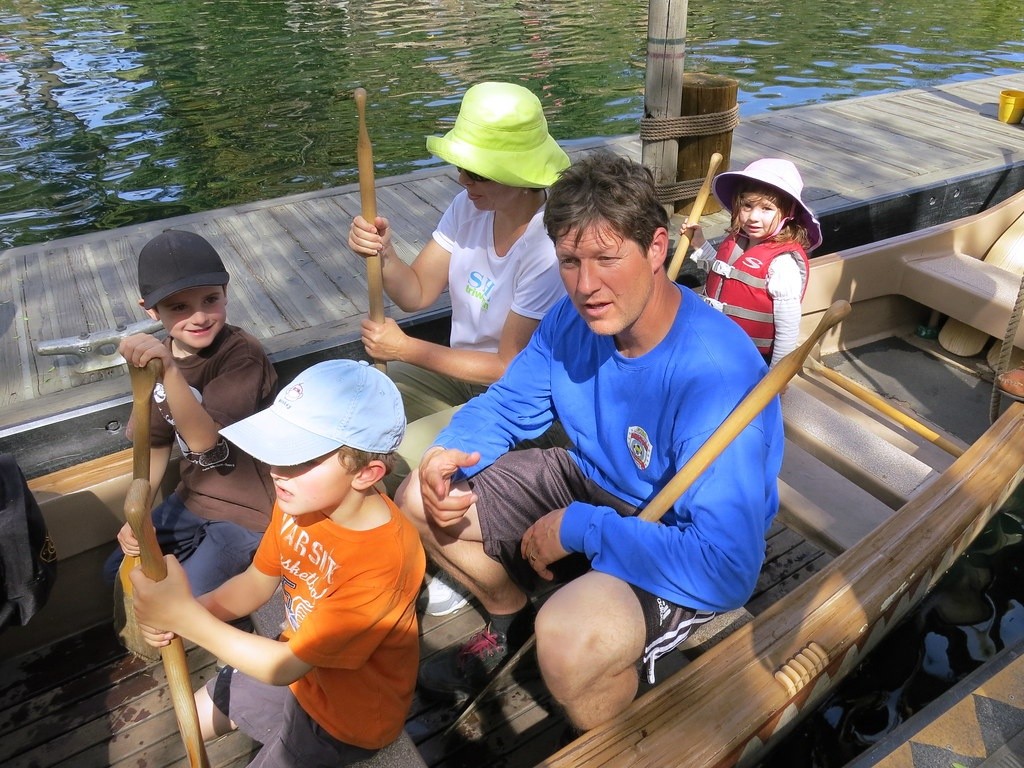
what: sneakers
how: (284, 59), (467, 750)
(418, 568), (475, 617)
(418, 620), (539, 693)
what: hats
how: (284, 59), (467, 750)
(217, 358), (407, 466)
(425, 82), (571, 189)
(137, 228), (229, 310)
(712, 158), (823, 253)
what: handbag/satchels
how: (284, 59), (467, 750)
(0, 453), (57, 634)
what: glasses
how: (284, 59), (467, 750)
(457, 165), (490, 182)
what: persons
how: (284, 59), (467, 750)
(348, 80), (570, 617)
(129, 359), (426, 768)
(396, 156), (783, 748)
(680, 158), (822, 396)
(102, 230), (279, 599)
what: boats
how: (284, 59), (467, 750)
(0, 195), (1024, 766)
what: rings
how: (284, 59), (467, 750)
(528, 552), (537, 562)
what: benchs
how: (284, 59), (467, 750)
(673, 604), (756, 663)
(250, 575), (427, 768)
(897, 252), (1024, 352)
(777, 382), (942, 511)
